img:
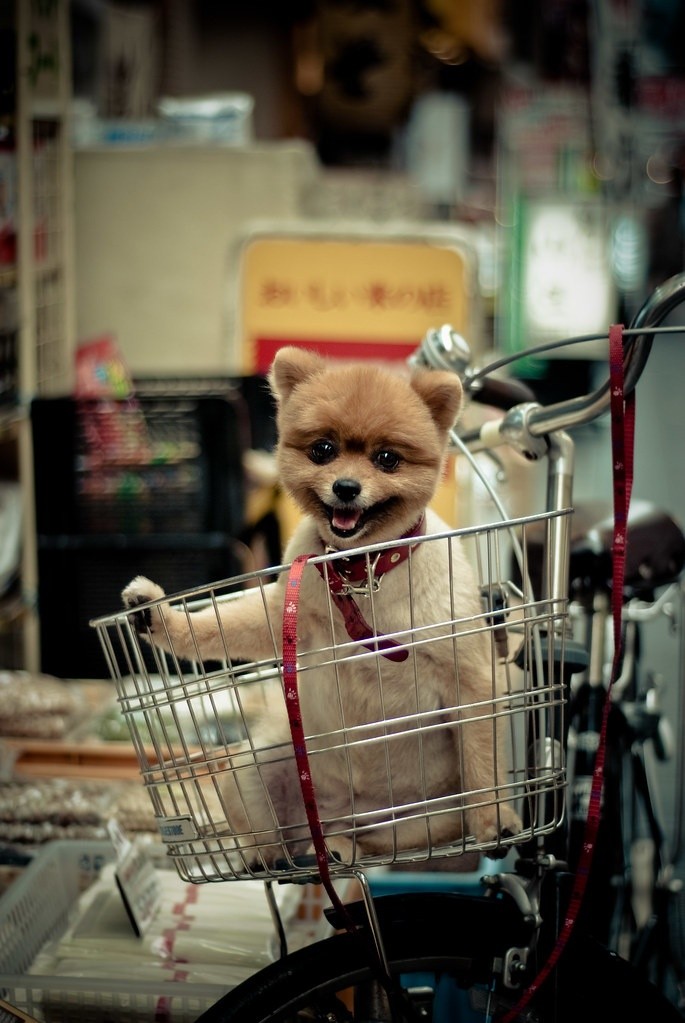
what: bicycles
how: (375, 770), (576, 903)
(88, 279), (685, 1023)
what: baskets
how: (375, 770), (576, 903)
(91, 506), (568, 883)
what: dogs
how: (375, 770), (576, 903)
(120, 347), (523, 863)
